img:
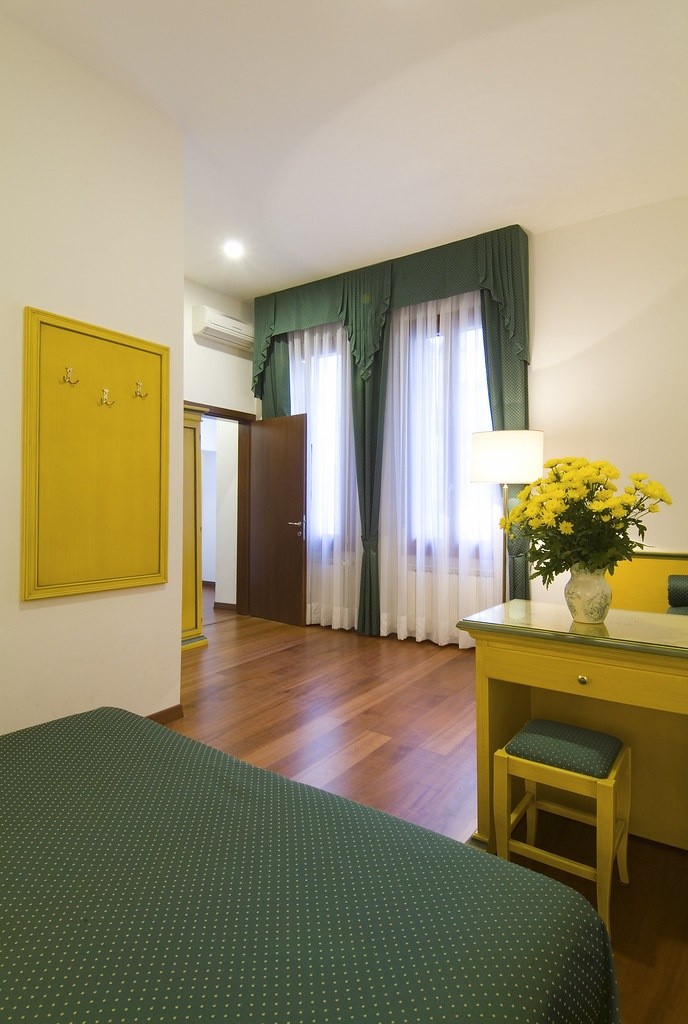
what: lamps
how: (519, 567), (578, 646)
(468, 430), (544, 604)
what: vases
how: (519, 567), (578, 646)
(564, 562), (612, 623)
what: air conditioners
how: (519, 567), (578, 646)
(192, 304), (254, 355)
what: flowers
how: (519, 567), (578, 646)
(499, 457), (672, 590)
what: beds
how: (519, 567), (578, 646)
(0, 704), (620, 1024)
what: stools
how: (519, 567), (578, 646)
(493, 717), (633, 940)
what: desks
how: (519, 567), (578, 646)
(456, 599), (688, 854)
